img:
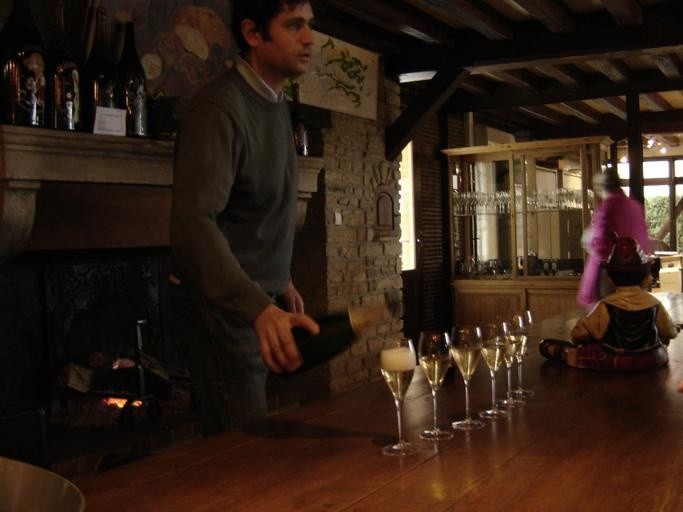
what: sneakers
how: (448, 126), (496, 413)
(538, 338), (573, 363)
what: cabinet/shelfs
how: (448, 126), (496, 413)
(441, 135), (615, 280)
(450, 280), (579, 328)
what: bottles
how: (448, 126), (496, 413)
(13, 15), (150, 140)
(284, 81), (314, 157)
(250, 298), (401, 380)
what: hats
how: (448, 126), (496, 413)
(591, 172), (618, 185)
(603, 238), (655, 272)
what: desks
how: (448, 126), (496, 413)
(71, 289), (683, 512)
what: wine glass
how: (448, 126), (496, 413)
(453, 185), (594, 214)
(380, 309), (541, 459)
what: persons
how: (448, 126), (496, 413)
(537, 229), (679, 373)
(572, 165), (652, 307)
(165, 1), (323, 439)
